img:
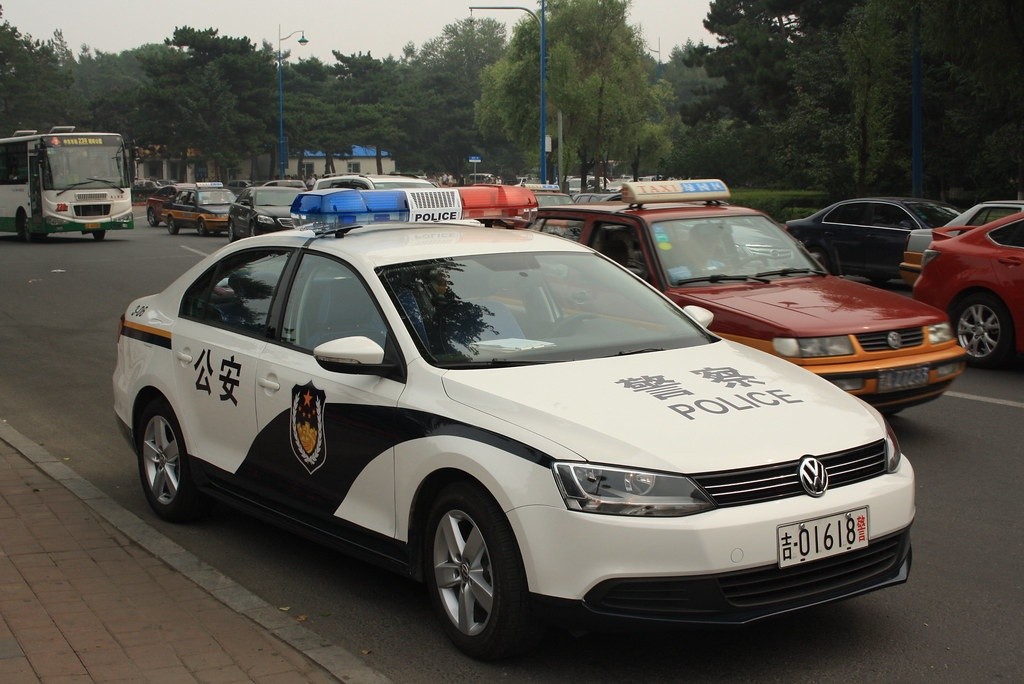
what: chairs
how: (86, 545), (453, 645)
(316, 283), (430, 360)
(438, 300), (525, 356)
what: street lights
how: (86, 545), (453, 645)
(468, 6), (547, 183)
(277, 24), (309, 179)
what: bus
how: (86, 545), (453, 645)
(0, 126), (139, 241)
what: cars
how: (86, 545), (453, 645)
(110, 181), (918, 664)
(226, 186), (305, 243)
(910, 207), (1024, 370)
(223, 172), (662, 233)
(783, 197), (963, 283)
(144, 182), (195, 228)
(899, 199), (1024, 291)
(160, 181), (238, 235)
(512, 177), (967, 412)
(225, 179), (255, 195)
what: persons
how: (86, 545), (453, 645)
(274, 171), (336, 189)
(604, 223), (766, 286)
(431, 168), (507, 187)
(2, 153), (111, 181)
